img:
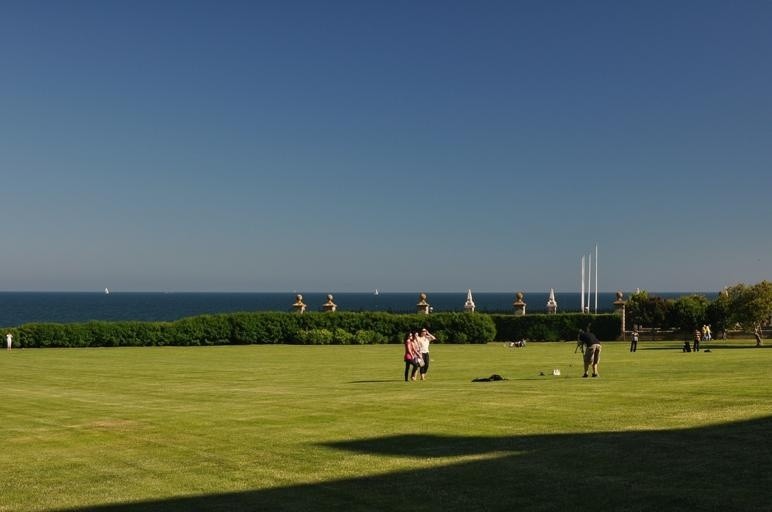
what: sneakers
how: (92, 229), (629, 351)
(583, 372), (599, 377)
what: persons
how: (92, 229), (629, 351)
(508, 338), (526, 348)
(681, 324), (713, 353)
(413, 331), (422, 380)
(577, 329), (600, 377)
(403, 331), (417, 382)
(629, 328), (639, 352)
(418, 293), (427, 304)
(5, 332), (13, 351)
(419, 328), (436, 380)
(513, 292), (523, 304)
(295, 294), (304, 305)
(575, 329), (585, 353)
(614, 290), (623, 302)
(327, 294), (334, 304)
